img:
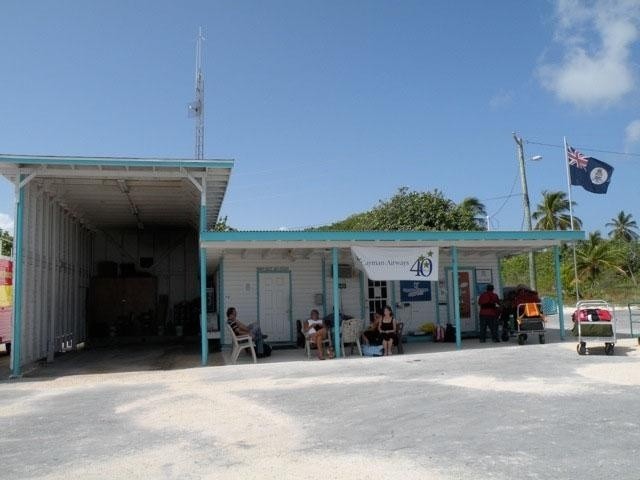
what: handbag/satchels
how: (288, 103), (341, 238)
(362, 344), (384, 357)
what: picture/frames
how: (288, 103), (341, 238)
(475, 269), (493, 284)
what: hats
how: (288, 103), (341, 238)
(487, 285), (495, 289)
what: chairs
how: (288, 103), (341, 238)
(226, 323), (256, 364)
(296, 313), (405, 359)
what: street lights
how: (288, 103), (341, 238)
(519, 155), (543, 291)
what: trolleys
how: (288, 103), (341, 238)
(501, 300), (547, 345)
(575, 297), (618, 355)
(627, 302), (640, 346)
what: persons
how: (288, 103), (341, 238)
(363, 308), (385, 345)
(479, 284), (503, 343)
(303, 309), (333, 360)
(227, 306), (271, 359)
(378, 304), (398, 355)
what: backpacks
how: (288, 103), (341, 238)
(444, 323), (456, 343)
(432, 323), (445, 342)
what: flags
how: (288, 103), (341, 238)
(564, 144), (614, 195)
